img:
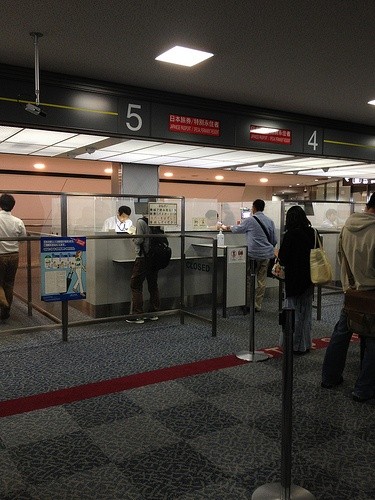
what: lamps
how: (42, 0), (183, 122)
(154, 27), (216, 67)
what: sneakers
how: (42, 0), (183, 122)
(143, 311), (158, 320)
(126, 316), (144, 323)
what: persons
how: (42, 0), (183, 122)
(0, 195), (26, 322)
(103, 206), (133, 233)
(216, 199), (277, 312)
(321, 191), (375, 401)
(203, 209), (231, 231)
(319, 208), (337, 227)
(126, 217), (159, 323)
(277, 205), (323, 357)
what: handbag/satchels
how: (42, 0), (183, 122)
(267, 258), (285, 280)
(310, 249), (333, 284)
(345, 288), (375, 336)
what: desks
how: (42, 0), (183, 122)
(192, 244), (248, 317)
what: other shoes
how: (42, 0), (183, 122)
(353, 395), (375, 402)
(321, 375), (343, 388)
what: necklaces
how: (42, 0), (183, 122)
(117, 224), (126, 231)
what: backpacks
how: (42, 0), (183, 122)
(136, 218), (171, 269)
